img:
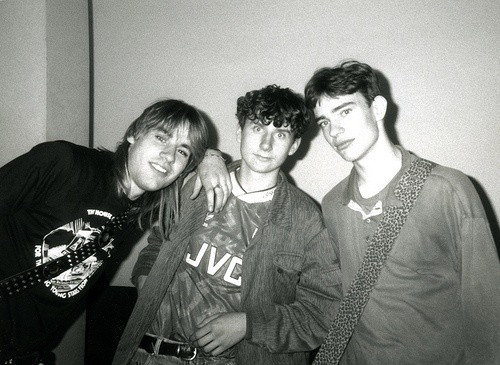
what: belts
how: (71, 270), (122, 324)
(137, 334), (208, 360)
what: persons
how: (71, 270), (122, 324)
(303, 60), (500, 365)
(0, 99), (231, 365)
(111, 84), (344, 365)
